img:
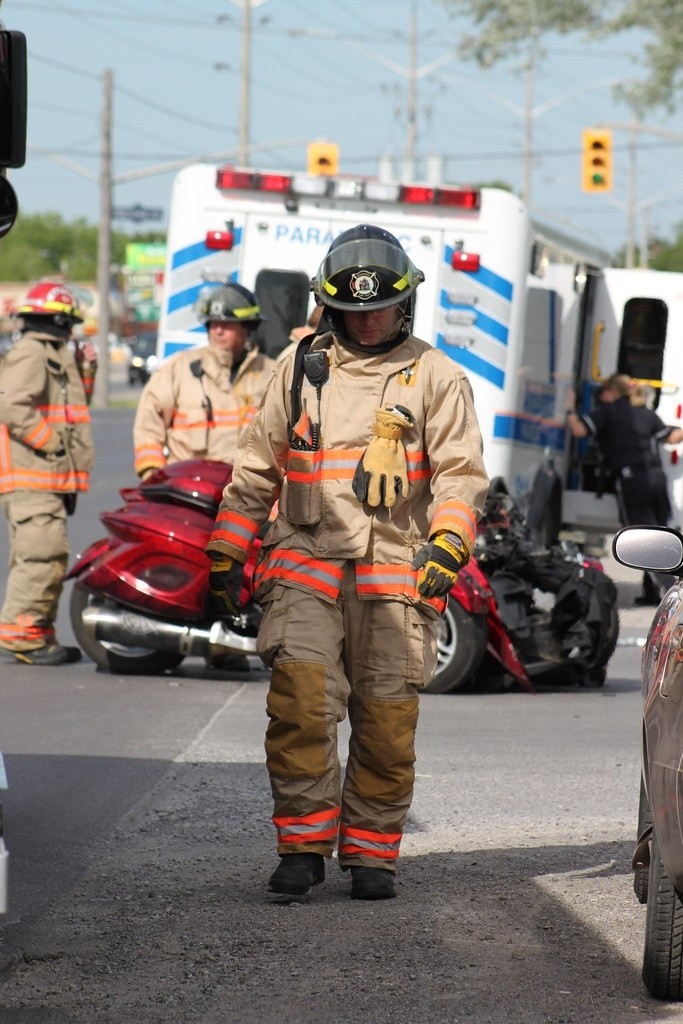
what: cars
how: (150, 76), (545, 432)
(129, 332), (158, 390)
(609, 525), (683, 1003)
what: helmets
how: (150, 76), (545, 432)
(9, 282), (85, 324)
(310, 224), (424, 310)
(198, 283), (267, 325)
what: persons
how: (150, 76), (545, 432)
(135, 283), (280, 672)
(204, 224), (487, 900)
(0, 284), (95, 667)
(565, 372), (682, 603)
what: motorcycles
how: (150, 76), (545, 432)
(58, 458), (499, 696)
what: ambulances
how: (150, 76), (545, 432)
(151, 160), (683, 547)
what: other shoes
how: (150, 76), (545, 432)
(209, 654), (250, 672)
(0, 644), (82, 665)
(636, 593), (660, 606)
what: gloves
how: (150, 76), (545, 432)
(352, 408), (414, 507)
(211, 553), (242, 618)
(411, 532), (468, 598)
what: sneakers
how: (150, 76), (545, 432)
(267, 852), (325, 895)
(348, 866), (397, 900)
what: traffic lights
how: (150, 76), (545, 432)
(579, 127), (618, 195)
(311, 145), (345, 181)
